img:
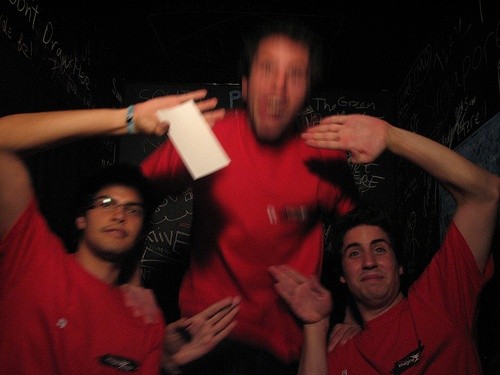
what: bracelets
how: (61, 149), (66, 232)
(126, 104), (136, 133)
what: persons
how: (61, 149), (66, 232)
(267, 113), (500, 375)
(0, 89), (242, 375)
(138, 25), (362, 375)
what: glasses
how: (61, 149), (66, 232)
(87, 197), (145, 219)
